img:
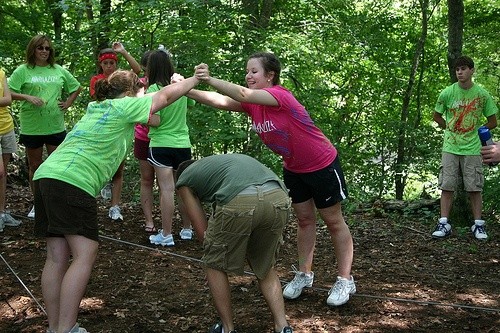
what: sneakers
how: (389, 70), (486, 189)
(471, 219), (488, 240)
(326, 275), (357, 306)
(282, 264), (314, 300)
(432, 216), (452, 239)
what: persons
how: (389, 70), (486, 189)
(134, 50), (156, 233)
(432, 56), (499, 240)
(31, 66), (204, 333)
(480, 142), (500, 163)
(90, 41), (141, 221)
(170, 52), (357, 306)
(7, 34), (83, 218)
(144, 48), (196, 247)
(175, 153), (294, 333)
(0, 68), (22, 232)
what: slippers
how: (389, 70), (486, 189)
(142, 223), (157, 233)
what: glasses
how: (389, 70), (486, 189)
(37, 45), (51, 51)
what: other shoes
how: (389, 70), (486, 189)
(157, 228), (163, 233)
(69, 322), (90, 333)
(179, 228), (193, 240)
(0, 213), (23, 227)
(108, 205), (123, 222)
(211, 323), (237, 333)
(149, 233), (175, 247)
(0, 218), (6, 232)
(280, 326), (293, 333)
(27, 205), (35, 218)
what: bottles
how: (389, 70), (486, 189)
(478, 125), (499, 166)
